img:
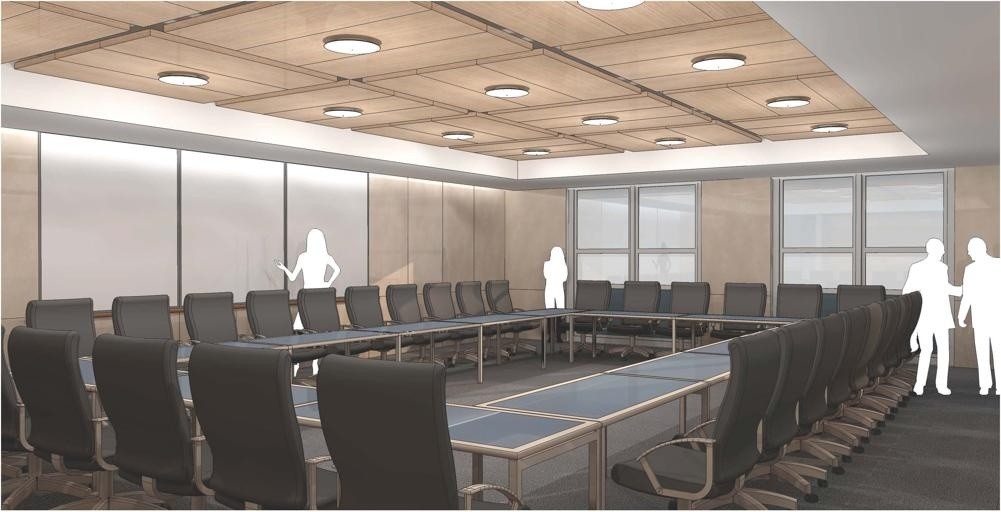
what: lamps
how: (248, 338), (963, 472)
(691, 51), (750, 76)
(321, 33), (383, 57)
(155, 68), (211, 88)
(582, 113), (689, 150)
(767, 95), (851, 135)
(322, 105), (365, 121)
(441, 127), (552, 160)
(482, 85), (530, 100)
